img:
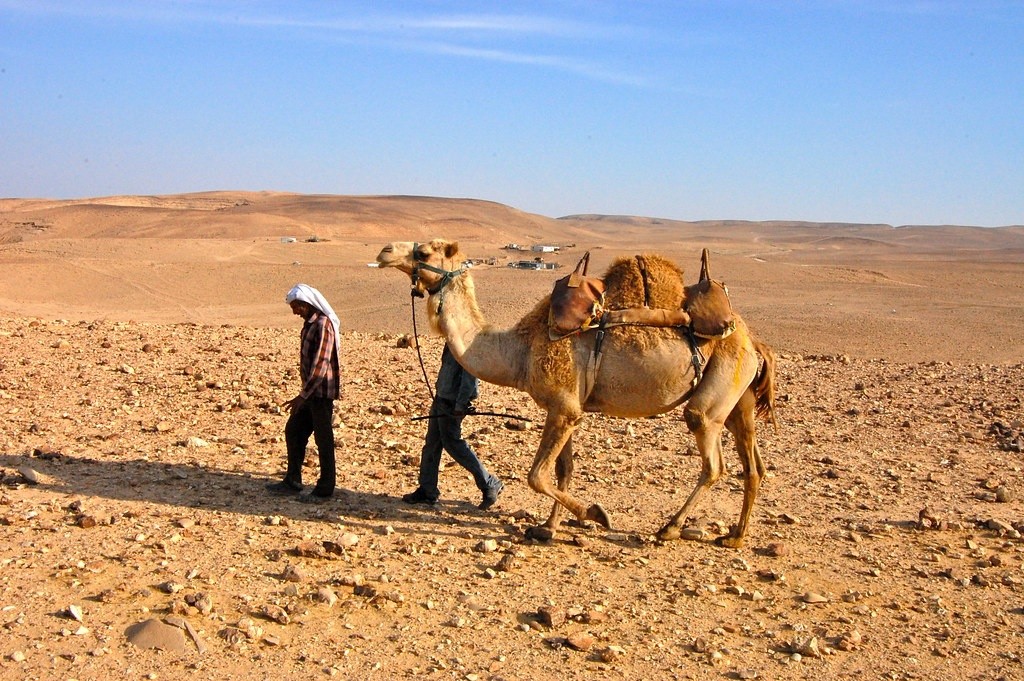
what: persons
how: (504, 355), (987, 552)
(402, 341), (505, 511)
(264, 284), (336, 504)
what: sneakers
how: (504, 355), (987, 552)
(296, 493), (330, 504)
(403, 487), (438, 504)
(265, 478), (301, 496)
(478, 481), (504, 510)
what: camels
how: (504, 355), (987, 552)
(378, 238), (778, 548)
